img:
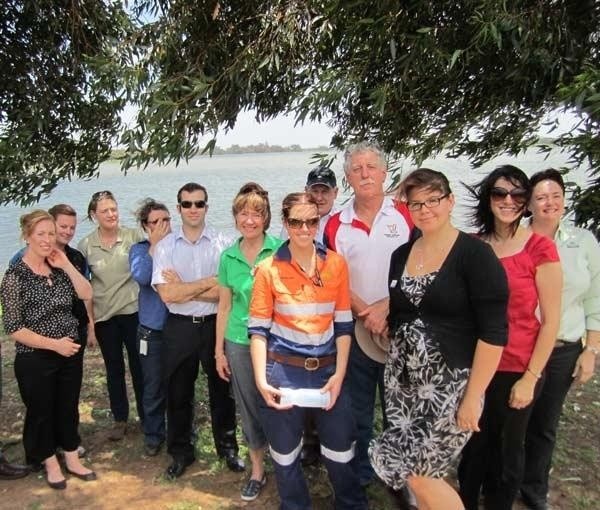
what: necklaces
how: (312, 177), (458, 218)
(101, 230), (115, 249)
(414, 238), (453, 271)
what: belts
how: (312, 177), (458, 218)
(267, 353), (337, 368)
(170, 313), (216, 324)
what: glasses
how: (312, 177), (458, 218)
(407, 195), (447, 211)
(286, 218), (320, 230)
(93, 192), (111, 200)
(490, 187), (529, 203)
(182, 201), (205, 208)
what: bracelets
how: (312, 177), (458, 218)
(584, 346), (599, 360)
(214, 350), (223, 360)
(527, 366), (541, 380)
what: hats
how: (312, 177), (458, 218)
(355, 319), (389, 364)
(308, 166), (336, 190)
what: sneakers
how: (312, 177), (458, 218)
(1, 440), (96, 489)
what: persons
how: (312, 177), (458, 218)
(246, 194), (369, 508)
(10, 203), (89, 473)
(280, 167), (339, 247)
(322, 141), (415, 510)
(214, 181), (284, 502)
(519, 168), (600, 510)
(151, 183), (246, 482)
(457, 165), (563, 509)
(79, 190), (145, 443)
(366, 168), (508, 509)
(127, 198), (198, 456)
(0, 342), (31, 481)
(1, 210), (97, 490)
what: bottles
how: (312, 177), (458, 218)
(279, 388), (332, 409)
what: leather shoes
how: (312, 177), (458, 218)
(108, 419), (318, 500)
